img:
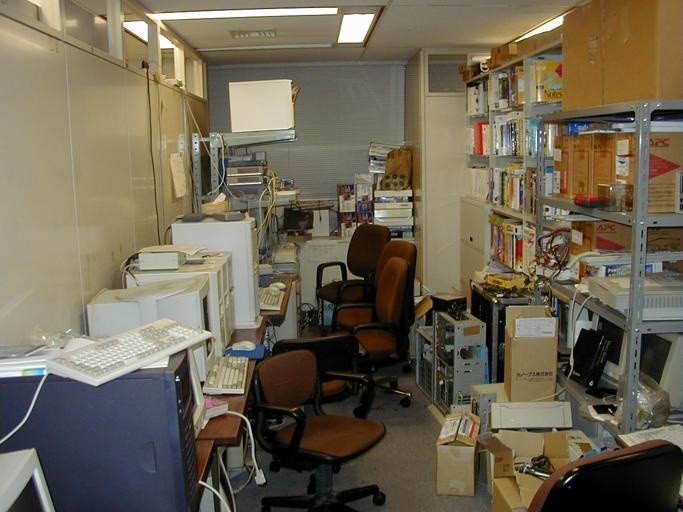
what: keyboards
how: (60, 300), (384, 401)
(203, 355), (249, 397)
(257, 286), (285, 311)
(48, 317), (214, 387)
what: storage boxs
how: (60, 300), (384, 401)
(428, 304), (600, 512)
(568, 220), (682, 272)
(552, 131), (682, 212)
(559, 1), (683, 112)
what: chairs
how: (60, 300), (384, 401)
(331, 256), (415, 419)
(271, 332), (360, 405)
(329, 239), (422, 332)
(253, 349), (386, 512)
(511, 439), (681, 512)
(315, 223), (390, 307)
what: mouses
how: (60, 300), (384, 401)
(230, 340), (256, 351)
(269, 282), (287, 292)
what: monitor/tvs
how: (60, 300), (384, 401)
(592, 312), (628, 382)
(187, 350), (207, 439)
(0, 447), (55, 512)
(640, 333), (683, 409)
(84, 273), (216, 383)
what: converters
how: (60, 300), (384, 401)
(182, 212), (207, 223)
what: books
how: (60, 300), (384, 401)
(222, 152), (267, 191)
(1, 343), (56, 378)
(372, 186), (415, 239)
(462, 61), (564, 274)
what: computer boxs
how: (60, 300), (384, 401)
(171, 218), (262, 330)
(0, 350), (201, 511)
(126, 264), (226, 357)
(184, 248), (235, 335)
(182, 260), (231, 346)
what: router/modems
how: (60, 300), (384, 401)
(214, 211), (245, 222)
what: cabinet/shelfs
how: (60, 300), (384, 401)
(489, 56), (556, 284)
(458, 196), (489, 285)
(540, 99), (682, 450)
(404, 49), (488, 296)
(460, 70), (489, 200)
